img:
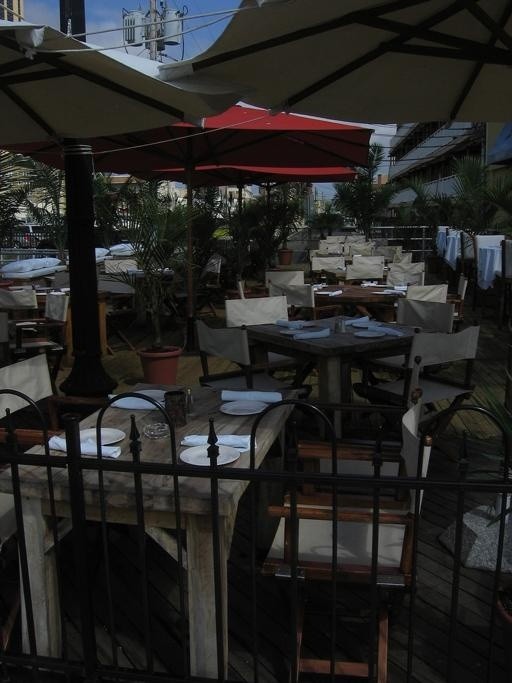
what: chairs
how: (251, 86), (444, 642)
(195, 228), (512, 438)
(1, 257), (69, 355)
(96, 244), (194, 353)
(2, 354), (433, 682)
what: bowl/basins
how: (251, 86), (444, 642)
(143, 423), (171, 439)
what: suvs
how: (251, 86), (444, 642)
(5, 219), (52, 249)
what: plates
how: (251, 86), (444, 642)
(134, 389), (168, 403)
(179, 444), (241, 467)
(353, 331), (385, 337)
(79, 427), (126, 447)
(280, 329), (311, 335)
(219, 398), (269, 416)
(298, 319), (315, 327)
(352, 321), (383, 329)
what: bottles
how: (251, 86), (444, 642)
(185, 388), (195, 417)
